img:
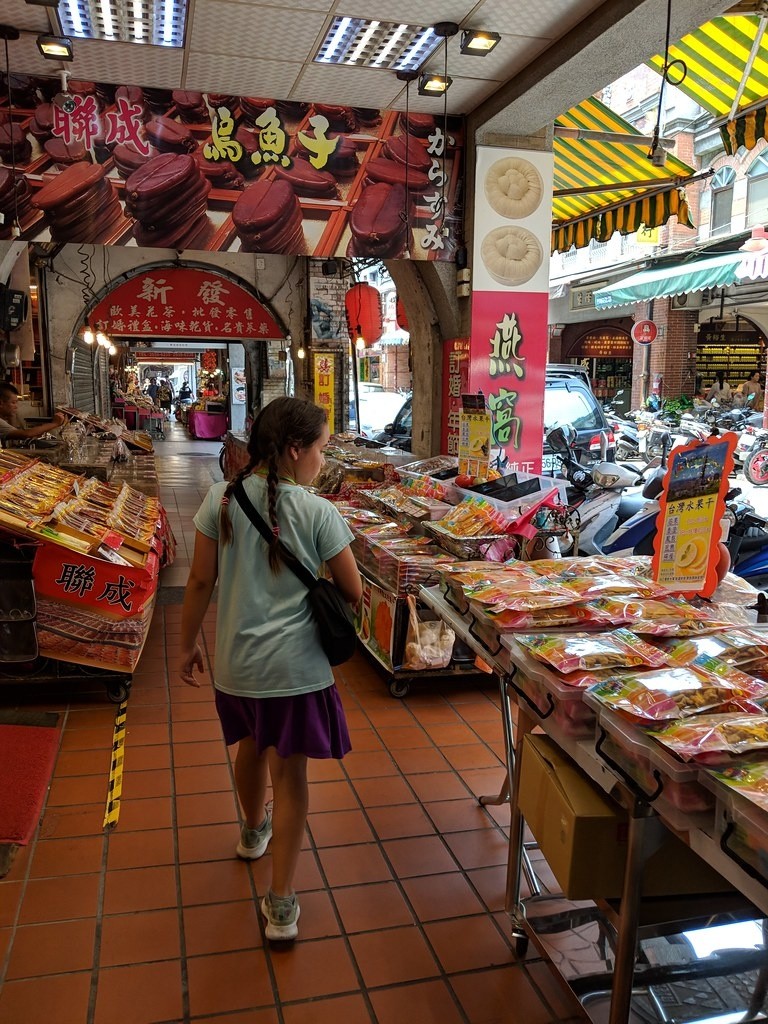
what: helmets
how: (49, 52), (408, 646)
(545, 419), (578, 452)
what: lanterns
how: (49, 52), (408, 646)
(345, 282), (382, 349)
(396, 293), (410, 332)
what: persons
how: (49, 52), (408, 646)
(174, 396), (363, 940)
(147, 375), (173, 422)
(706, 370), (732, 406)
(179, 382), (194, 405)
(743, 371), (762, 412)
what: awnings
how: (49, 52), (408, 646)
(592, 258), (767, 313)
(553, 13), (768, 256)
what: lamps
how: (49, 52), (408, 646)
(36, 35), (74, 62)
(345, 279), (383, 349)
(418, 73), (453, 97)
(460, 29), (502, 57)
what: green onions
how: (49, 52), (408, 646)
(41, 530), (80, 547)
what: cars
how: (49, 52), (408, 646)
(370, 363), (616, 481)
(349, 381), (412, 440)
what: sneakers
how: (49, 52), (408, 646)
(235, 799), (274, 861)
(260, 887), (301, 939)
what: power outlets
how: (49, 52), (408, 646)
(256, 259), (265, 269)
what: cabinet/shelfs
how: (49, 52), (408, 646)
(224, 420), (558, 593)
(696, 344), (762, 387)
(114, 378), (163, 418)
(188, 371), (226, 412)
(0, 405), (162, 573)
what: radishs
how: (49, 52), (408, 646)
(57, 532), (90, 547)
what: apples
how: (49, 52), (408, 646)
(455, 473), (500, 488)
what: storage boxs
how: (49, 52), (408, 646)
(328, 454), (571, 597)
(510, 736), (736, 903)
(441, 567), (768, 884)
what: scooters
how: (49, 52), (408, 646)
(603, 391), (768, 485)
(547, 421), (768, 615)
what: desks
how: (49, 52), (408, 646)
(420, 589), (766, 1024)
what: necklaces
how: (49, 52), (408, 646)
(256, 466), (301, 485)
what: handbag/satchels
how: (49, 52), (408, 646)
(304, 577), (357, 667)
